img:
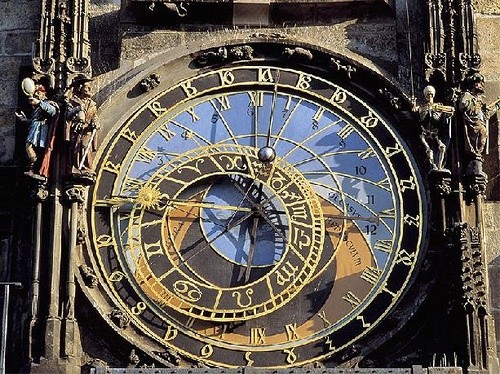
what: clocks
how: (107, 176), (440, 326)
(74, 32), (447, 374)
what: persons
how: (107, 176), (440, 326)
(63, 74), (101, 176)
(16, 80), (59, 173)
(457, 71), (499, 179)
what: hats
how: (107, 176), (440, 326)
(70, 73), (93, 87)
